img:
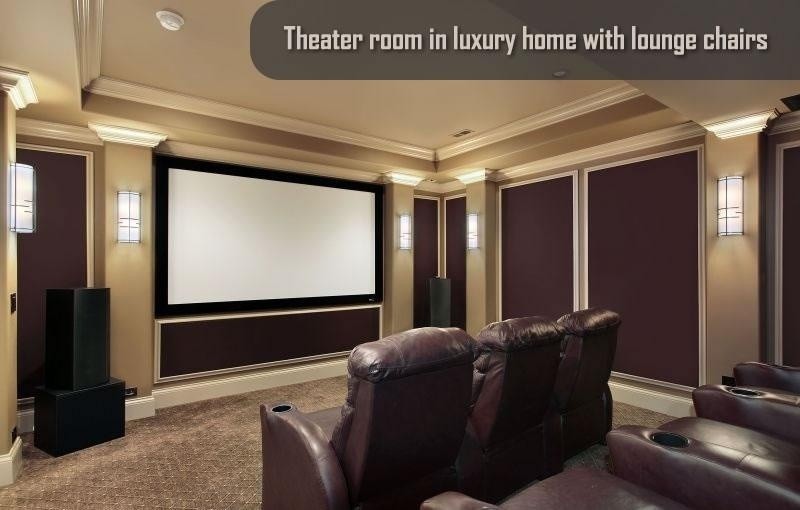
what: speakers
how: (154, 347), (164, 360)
(33, 377), (125, 458)
(42, 288), (111, 392)
(429, 279), (451, 328)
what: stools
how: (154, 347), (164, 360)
(419, 356), (800, 508)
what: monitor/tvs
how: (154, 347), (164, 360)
(155, 155), (384, 317)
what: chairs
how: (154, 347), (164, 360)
(254, 300), (624, 510)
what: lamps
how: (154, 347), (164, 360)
(5, 160), (40, 239)
(115, 188), (142, 246)
(713, 175), (746, 237)
(463, 209), (481, 251)
(398, 207), (412, 255)
